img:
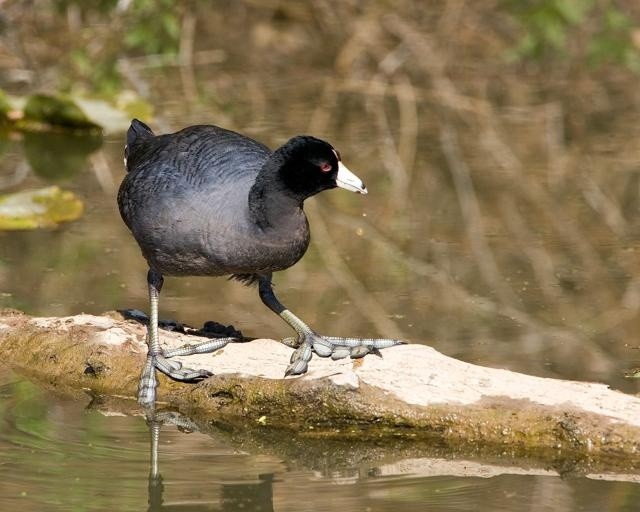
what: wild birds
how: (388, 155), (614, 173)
(116, 117), (407, 411)
